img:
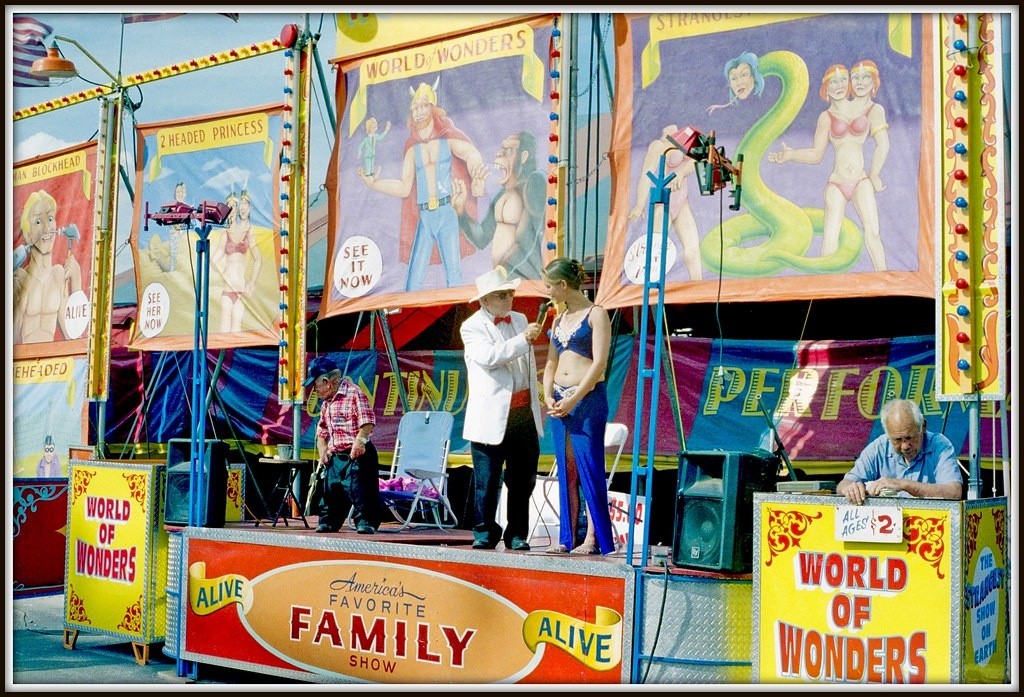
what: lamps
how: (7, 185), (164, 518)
(663, 125), (728, 197)
(29, 37), (121, 87)
(158, 202), (234, 228)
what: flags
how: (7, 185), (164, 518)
(123, 13), (239, 24)
(13, 16), (54, 89)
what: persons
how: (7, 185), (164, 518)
(459, 269), (544, 550)
(542, 257), (615, 556)
(837, 399), (964, 506)
(302, 356), (384, 534)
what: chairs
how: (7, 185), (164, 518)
(347, 411), (458, 532)
(524, 423), (630, 545)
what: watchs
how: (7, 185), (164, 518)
(356, 436), (367, 444)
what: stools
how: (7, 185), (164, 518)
(254, 457), (311, 529)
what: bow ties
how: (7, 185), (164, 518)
(494, 315), (511, 326)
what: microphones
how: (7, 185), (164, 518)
(529, 303), (549, 343)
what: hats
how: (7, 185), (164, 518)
(468, 265), (522, 304)
(302, 357), (338, 387)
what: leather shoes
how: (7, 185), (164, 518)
(472, 531), (495, 549)
(505, 537), (530, 549)
(357, 520), (374, 533)
(316, 524), (339, 532)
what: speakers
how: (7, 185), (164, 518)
(163, 442), (231, 529)
(671, 452), (778, 575)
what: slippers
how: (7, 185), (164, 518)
(571, 544), (599, 555)
(545, 545), (567, 553)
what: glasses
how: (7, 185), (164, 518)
(490, 289), (515, 300)
